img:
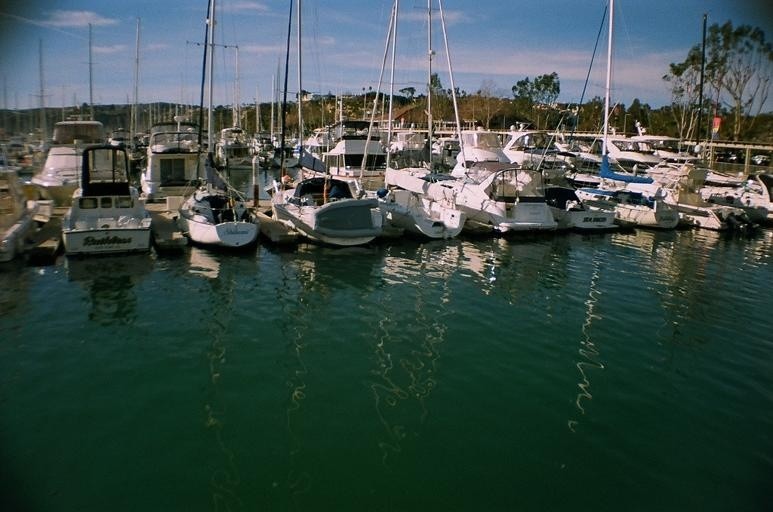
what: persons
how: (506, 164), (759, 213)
(137, 167), (152, 200)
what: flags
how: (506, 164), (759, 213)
(711, 116), (721, 141)
